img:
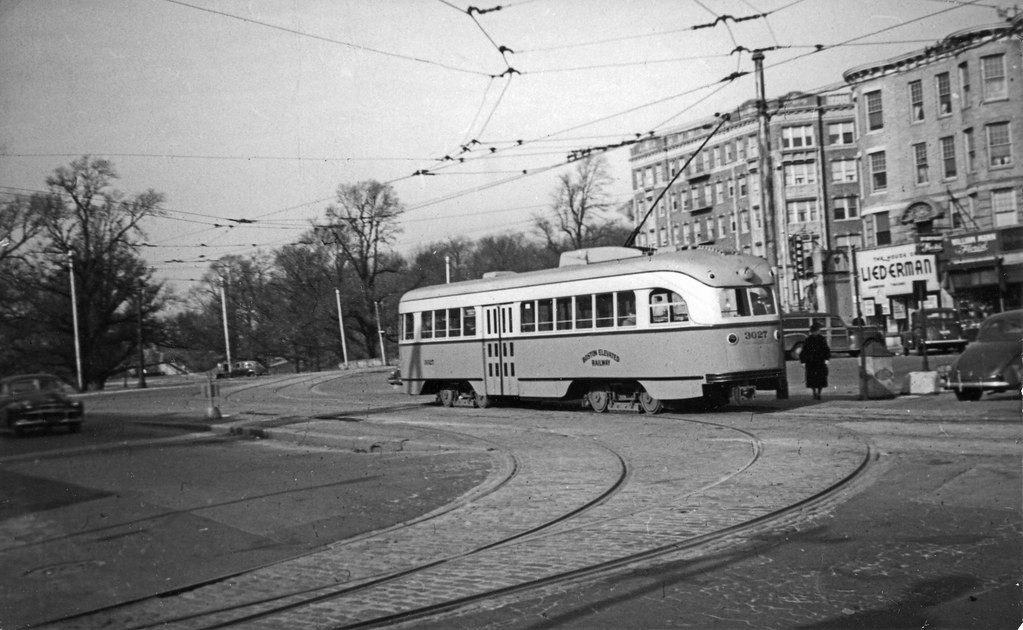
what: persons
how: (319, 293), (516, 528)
(852, 311), (866, 326)
(620, 304), (637, 326)
(799, 322), (831, 400)
(748, 290), (770, 315)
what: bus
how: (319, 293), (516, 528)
(396, 244), (787, 413)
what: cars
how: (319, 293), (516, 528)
(900, 310), (969, 355)
(947, 309), (1022, 401)
(1, 371), (86, 437)
(227, 360), (270, 376)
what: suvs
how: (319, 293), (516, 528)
(769, 311), (889, 359)
